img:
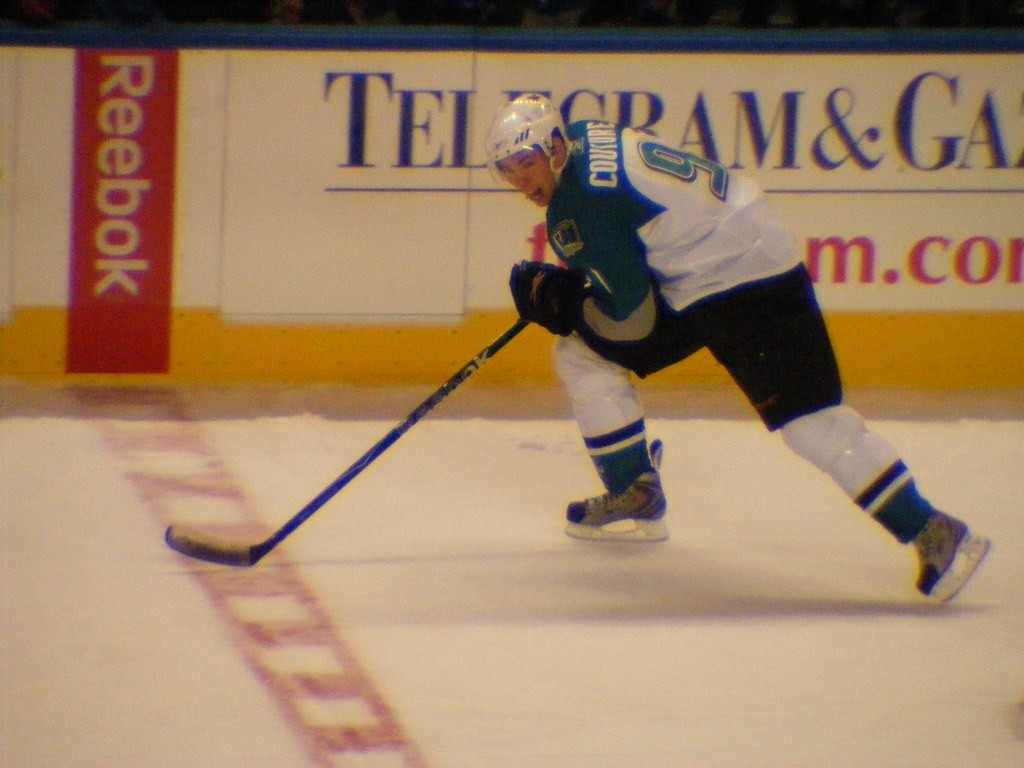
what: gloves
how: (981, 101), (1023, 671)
(509, 259), (586, 337)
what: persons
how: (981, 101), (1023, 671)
(486, 93), (968, 596)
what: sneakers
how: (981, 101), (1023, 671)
(559, 439), (670, 542)
(911, 508), (991, 604)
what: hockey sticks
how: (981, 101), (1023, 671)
(162, 317), (532, 569)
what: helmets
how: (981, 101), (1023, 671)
(481, 92), (567, 163)
